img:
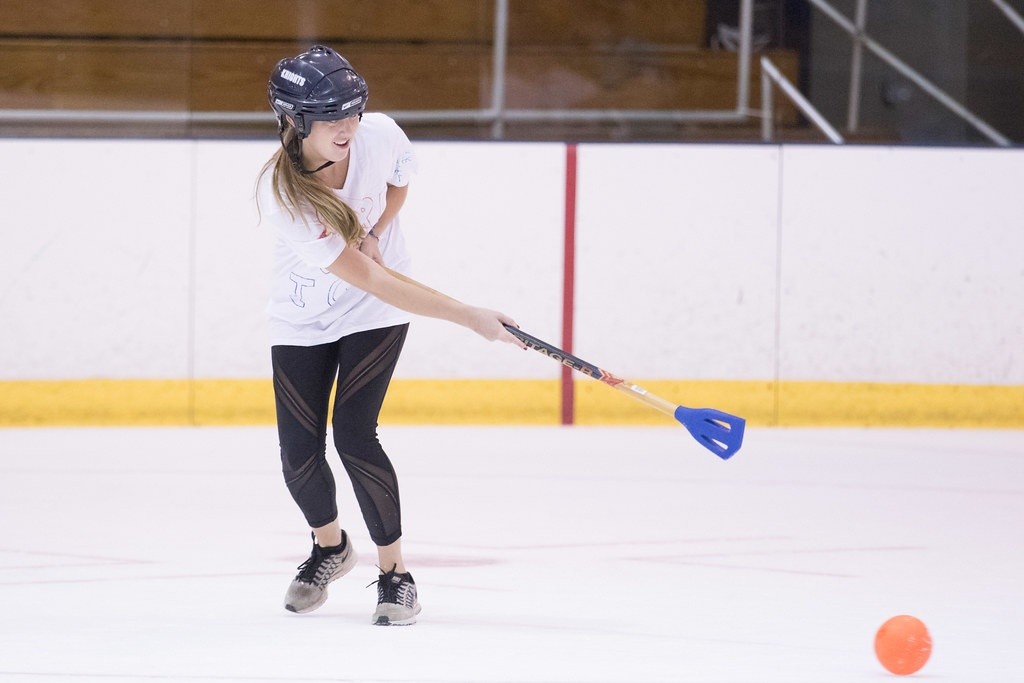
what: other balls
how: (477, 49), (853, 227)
(873, 613), (935, 677)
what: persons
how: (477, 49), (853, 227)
(254, 43), (527, 625)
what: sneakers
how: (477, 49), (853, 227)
(366, 562), (421, 626)
(283, 529), (358, 614)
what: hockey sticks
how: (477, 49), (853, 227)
(383, 264), (747, 463)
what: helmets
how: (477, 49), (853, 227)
(266, 45), (369, 139)
